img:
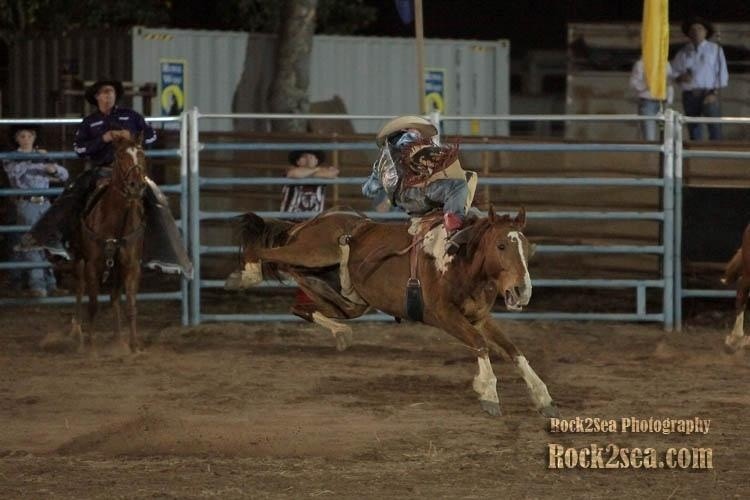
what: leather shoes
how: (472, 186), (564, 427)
(444, 224), (475, 257)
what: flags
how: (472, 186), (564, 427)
(639, 0), (671, 102)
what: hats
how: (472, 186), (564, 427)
(84, 78), (126, 107)
(681, 12), (716, 40)
(287, 148), (326, 168)
(375, 114), (440, 148)
(8, 123), (41, 147)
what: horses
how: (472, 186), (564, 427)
(719, 224), (750, 355)
(66, 129), (148, 355)
(223, 202), (563, 419)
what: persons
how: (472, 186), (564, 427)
(676, 20), (729, 142)
(277, 149), (341, 313)
(21, 76), (195, 280)
(628, 50), (677, 142)
(359, 115), (483, 235)
(3, 125), (70, 298)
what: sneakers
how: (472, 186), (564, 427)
(29, 284), (70, 298)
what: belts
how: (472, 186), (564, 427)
(15, 194), (52, 205)
(640, 97), (668, 104)
